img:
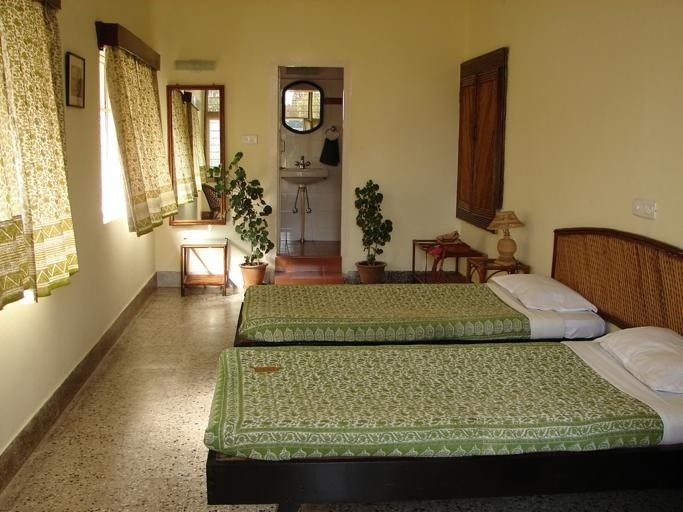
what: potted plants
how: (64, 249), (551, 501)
(203, 151), (275, 289)
(354, 178), (393, 284)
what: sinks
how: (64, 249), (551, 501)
(279, 167), (329, 184)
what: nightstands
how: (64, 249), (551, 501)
(466, 256), (529, 283)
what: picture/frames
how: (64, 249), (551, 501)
(65, 51), (87, 108)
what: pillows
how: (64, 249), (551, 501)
(592, 326), (682, 396)
(490, 272), (598, 313)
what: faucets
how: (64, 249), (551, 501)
(299, 155), (305, 169)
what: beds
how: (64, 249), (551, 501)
(203, 324), (683, 504)
(233, 282), (622, 345)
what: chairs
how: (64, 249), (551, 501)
(202, 183), (225, 220)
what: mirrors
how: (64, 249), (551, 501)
(282, 80), (324, 135)
(166, 84), (226, 225)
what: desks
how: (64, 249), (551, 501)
(178, 238), (230, 297)
(411, 238), (485, 284)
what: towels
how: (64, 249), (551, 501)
(320, 137), (340, 166)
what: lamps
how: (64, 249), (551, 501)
(485, 211), (525, 266)
